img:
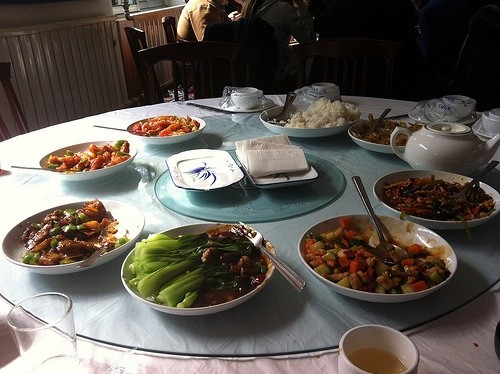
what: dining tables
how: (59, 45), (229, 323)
(0, 93), (500, 374)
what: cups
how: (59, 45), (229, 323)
(336, 324), (421, 374)
(7, 292), (80, 374)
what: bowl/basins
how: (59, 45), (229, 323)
(482, 108), (500, 134)
(442, 94), (477, 117)
(308, 82), (340, 101)
(230, 87), (263, 109)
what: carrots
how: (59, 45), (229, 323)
(304, 218), (428, 294)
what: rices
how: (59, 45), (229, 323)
(263, 96), (361, 128)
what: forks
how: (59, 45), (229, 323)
(231, 220), (306, 293)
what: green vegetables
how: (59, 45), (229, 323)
(400, 181), (496, 240)
(128, 232), (266, 308)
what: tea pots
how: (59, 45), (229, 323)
(389, 121), (500, 180)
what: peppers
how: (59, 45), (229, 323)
(23, 209), (130, 265)
(48, 140), (132, 172)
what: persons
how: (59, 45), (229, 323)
(305, 0), (500, 112)
(176, 0), (313, 95)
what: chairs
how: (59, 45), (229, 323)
(161, 15), (191, 100)
(124, 26), (163, 106)
(446, 4), (500, 112)
(291, 39), (399, 100)
(136, 39), (248, 106)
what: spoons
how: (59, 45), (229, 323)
(350, 175), (409, 264)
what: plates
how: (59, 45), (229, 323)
(165, 149), (318, 192)
(2, 200), (146, 275)
(222, 98), (274, 112)
(259, 106), (359, 138)
(128, 114), (207, 145)
(347, 120), (422, 155)
(120, 224), (278, 317)
(39, 141), (138, 180)
(372, 170), (500, 230)
(408, 111), (477, 124)
(471, 116), (493, 138)
(296, 214), (459, 304)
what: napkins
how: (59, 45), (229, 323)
(234, 133), (310, 178)
